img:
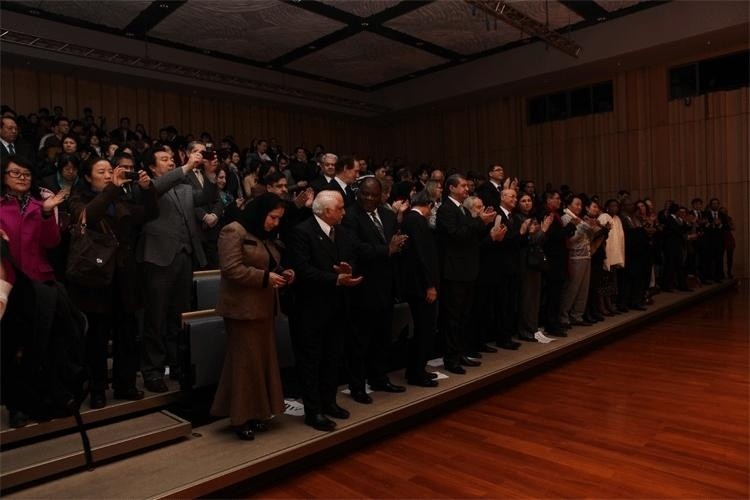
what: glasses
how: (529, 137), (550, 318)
(5, 169), (31, 180)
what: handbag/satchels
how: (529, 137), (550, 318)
(527, 236), (551, 273)
(590, 254), (608, 275)
(393, 303), (413, 340)
(67, 209), (119, 285)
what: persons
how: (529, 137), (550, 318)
(1, 107), (520, 444)
(482, 162), (736, 345)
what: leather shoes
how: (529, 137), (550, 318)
(235, 426), (254, 441)
(304, 405), (349, 431)
(351, 371), (438, 404)
(497, 334), (538, 350)
(445, 338), (497, 374)
(90, 378), (168, 409)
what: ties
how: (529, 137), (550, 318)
(371, 211), (385, 243)
(7, 143), (14, 156)
(509, 214), (513, 226)
(498, 186), (502, 191)
(714, 212), (716, 221)
(697, 210), (701, 219)
(329, 227), (335, 243)
(345, 185), (354, 201)
(123, 183), (130, 194)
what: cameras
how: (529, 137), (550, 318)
(199, 150), (216, 160)
(125, 171), (141, 181)
